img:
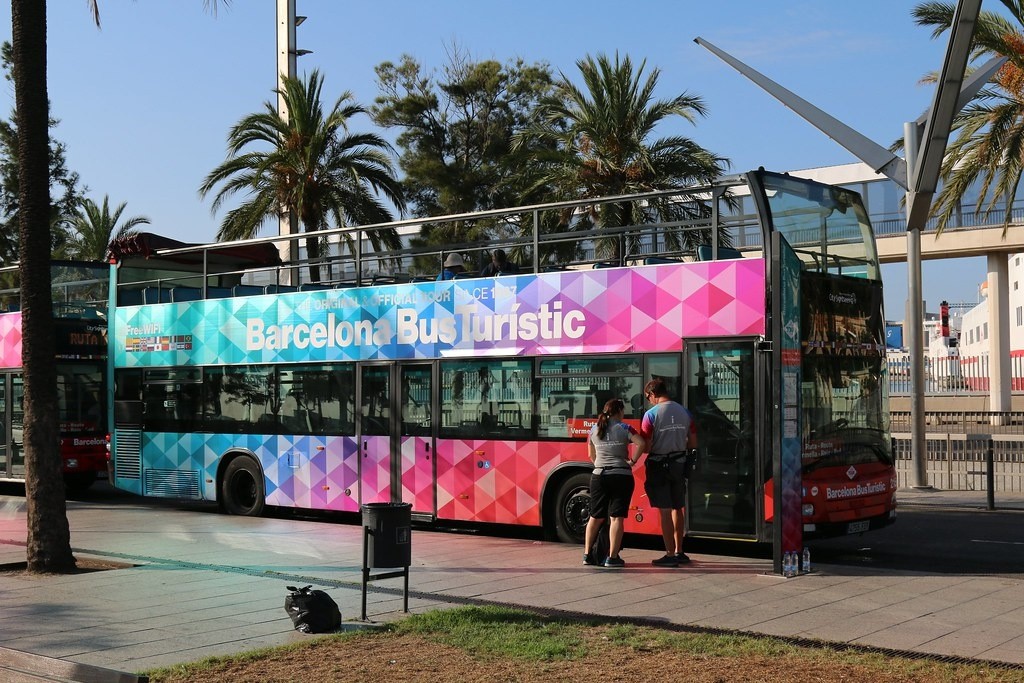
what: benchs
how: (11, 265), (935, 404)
(119, 245), (743, 436)
(0, 297), (108, 320)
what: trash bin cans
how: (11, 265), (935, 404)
(361, 503), (413, 568)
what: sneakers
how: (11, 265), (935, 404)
(651, 555), (679, 567)
(604, 554), (624, 566)
(582, 554), (596, 565)
(675, 552), (689, 563)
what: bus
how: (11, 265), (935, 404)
(1, 259), (111, 497)
(107, 165), (906, 560)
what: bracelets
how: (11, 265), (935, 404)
(630, 459), (637, 464)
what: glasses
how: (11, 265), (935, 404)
(646, 393), (651, 402)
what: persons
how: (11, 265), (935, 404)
(583, 400), (646, 566)
(558, 408), (573, 438)
(436, 253), (468, 281)
(640, 379), (698, 567)
(480, 250), (519, 277)
